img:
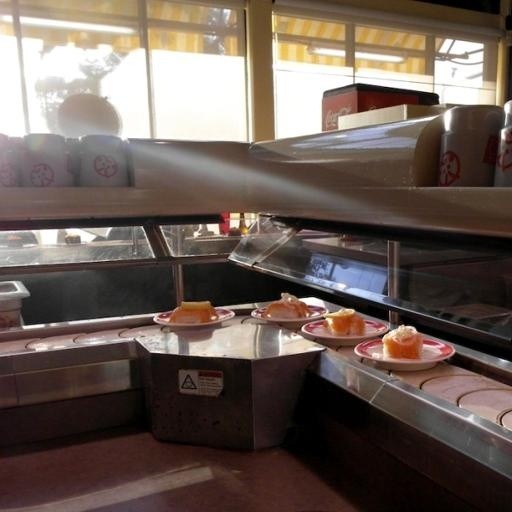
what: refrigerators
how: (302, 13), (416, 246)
(321, 83), (440, 132)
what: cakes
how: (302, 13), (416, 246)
(262, 292), (308, 318)
(167, 299), (217, 322)
(322, 306), (366, 336)
(380, 324), (424, 359)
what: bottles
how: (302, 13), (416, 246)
(238, 213), (248, 236)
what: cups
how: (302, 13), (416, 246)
(0, 134), (128, 186)
(438, 100), (512, 186)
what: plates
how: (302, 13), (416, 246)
(298, 317), (392, 340)
(248, 304), (329, 322)
(152, 308), (235, 328)
(352, 337), (455, 372)
